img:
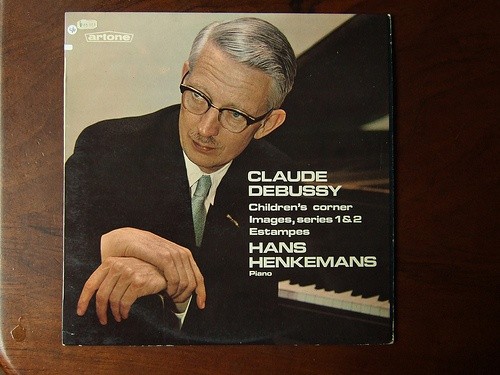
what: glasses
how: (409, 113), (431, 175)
(180, 71), (274, 134)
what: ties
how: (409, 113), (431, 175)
(191, 175), (213, 246)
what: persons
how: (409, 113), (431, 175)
(63, 18), (302, 346)
